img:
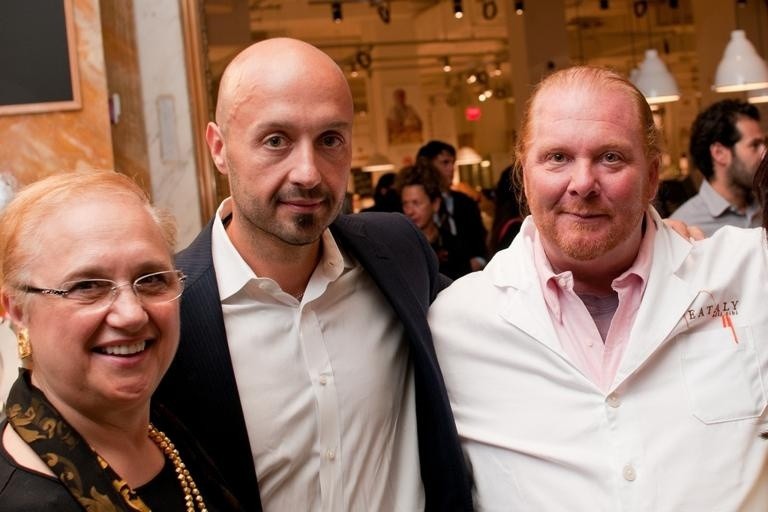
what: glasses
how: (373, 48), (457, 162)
(16, 271), (188, 311)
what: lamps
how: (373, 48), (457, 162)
(625, 0), (682, 109)
(743, 90), (767, 106)
(708, 0), (767, 94)
(620, 0), (640, 84)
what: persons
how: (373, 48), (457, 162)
(0, 168), (246, 512)
(146, 37), (707, 511)
(665, 96), (766, 239)
(357, 140), (524, 280)
(409, 65), (767, 511)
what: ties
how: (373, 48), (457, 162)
(437, 198), (451, 234)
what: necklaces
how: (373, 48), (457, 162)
(148, 421), (212, 510)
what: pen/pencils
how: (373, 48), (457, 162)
(721, 312), (738, 345)
